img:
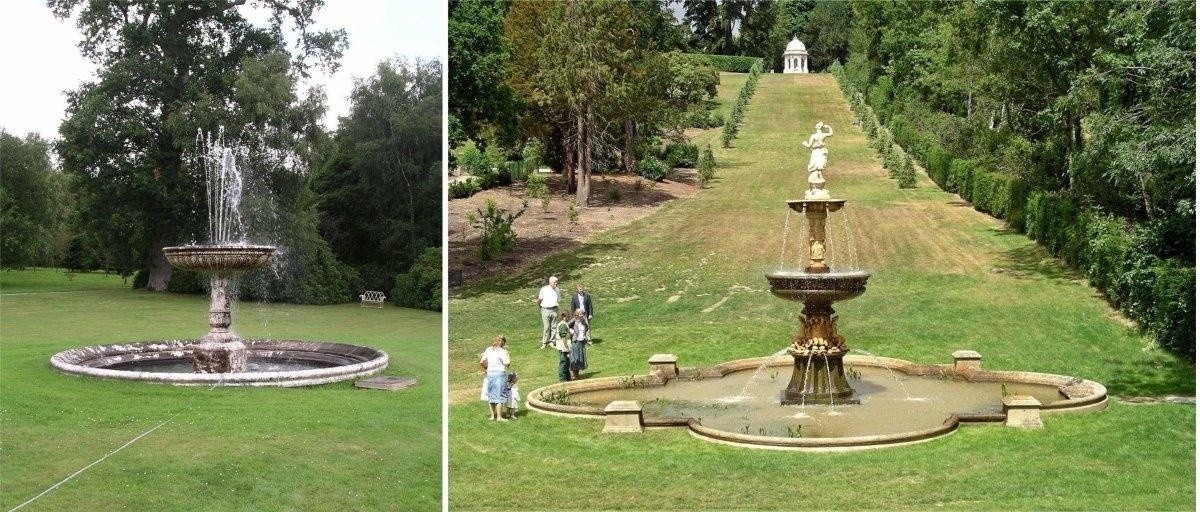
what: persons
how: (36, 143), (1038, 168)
(479, 335), (510, 422)
(568, 308), (590, 380)
(556, 310), (573, 382)
(537, 276), (561, 349)
(571, 282), (594, 345)
(505, 372), (521, 420)
(802, 122), (833, 174)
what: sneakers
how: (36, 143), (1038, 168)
(541, 342), (557, 350)
